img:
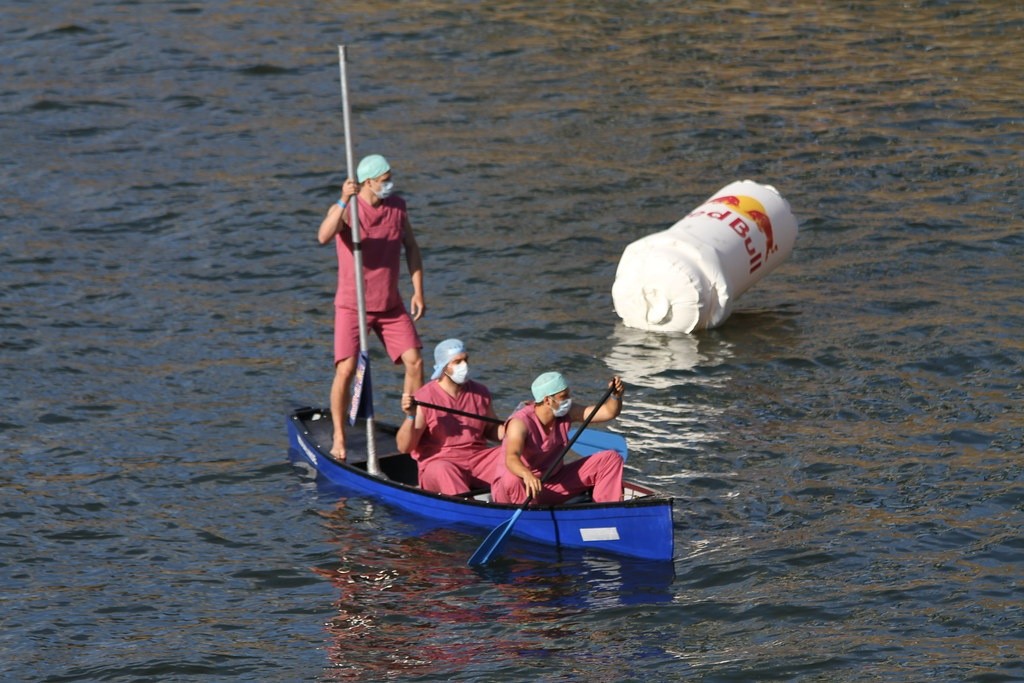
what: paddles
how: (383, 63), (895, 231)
(466, 378), (615, 567)
(413, 397), (504, 427)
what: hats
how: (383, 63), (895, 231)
(430, 339), (467, 378)
(531, 372), (568, 403)
(357, 154), (390, 184)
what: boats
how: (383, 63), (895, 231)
(282, 398), (677, 563)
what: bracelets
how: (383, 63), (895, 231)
(337, 199), (346, 208)
(407, 415), (415, 419)
(611, 394), (623, 402)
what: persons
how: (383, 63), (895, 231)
(319, 154), (425, 459)
(396, 339), (505, 495)
(491, 371), (625, 503)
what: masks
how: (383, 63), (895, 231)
(445, 360), (471, 384)
(370, 180), (394, 199)
(547, 396), (572, 417)
